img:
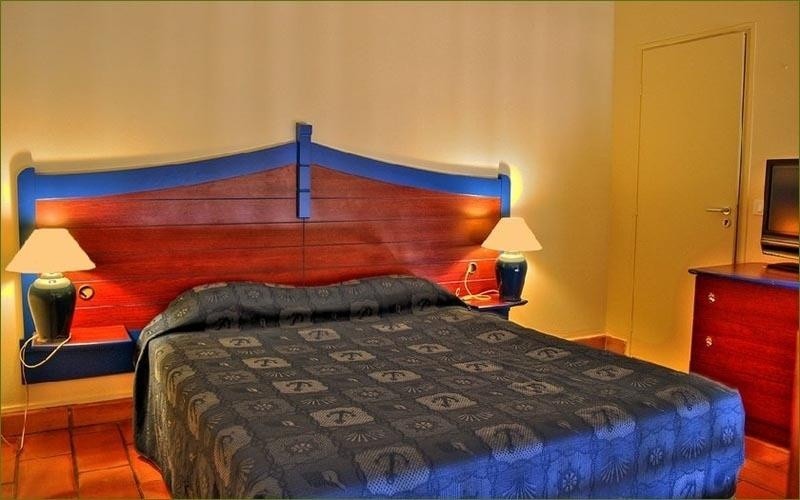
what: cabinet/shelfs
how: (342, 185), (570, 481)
(667, 256), (800, 451)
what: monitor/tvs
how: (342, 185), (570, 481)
(760, 159), (800, 273)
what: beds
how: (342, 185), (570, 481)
(13, 119), (747, 499)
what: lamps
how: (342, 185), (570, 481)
(478, 214), (542, 303)
(4, 225), (97, 343)
